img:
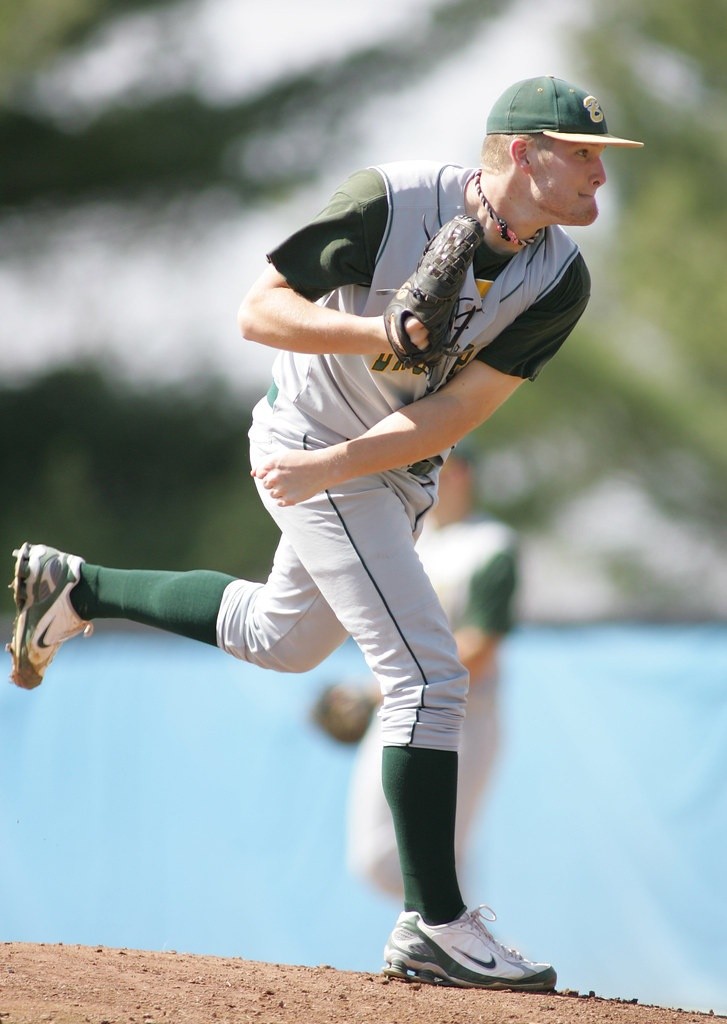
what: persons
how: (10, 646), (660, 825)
(3, 75), (647, 992)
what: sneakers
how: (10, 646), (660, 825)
(5, 541), (94, 690)
(382, 905), (556, 991)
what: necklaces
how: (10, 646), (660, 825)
(474, 168), (540, 246)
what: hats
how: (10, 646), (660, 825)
(485, 75), (645, 148)
(451, 427), (487, 463)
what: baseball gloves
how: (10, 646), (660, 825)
(374, 212), (496, 373)
